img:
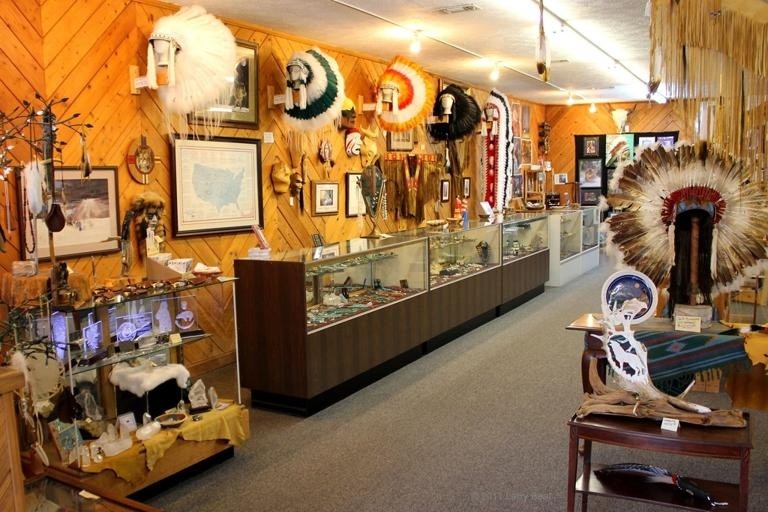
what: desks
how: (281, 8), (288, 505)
(567, 404), (754, 511)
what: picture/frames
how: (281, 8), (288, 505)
(168, 35), (265, 241)
(14, 163), (122, 265)
(311, 170), (368, 219)
(440, 176), (472, 204)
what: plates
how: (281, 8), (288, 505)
(154, 413), (187, 429)
(526, 204), (544, 210)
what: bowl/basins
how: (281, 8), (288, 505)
(478, 214), (490, 219)
(446, 217), (463, 225)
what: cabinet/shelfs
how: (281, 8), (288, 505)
(22, 275), (241, 498)
